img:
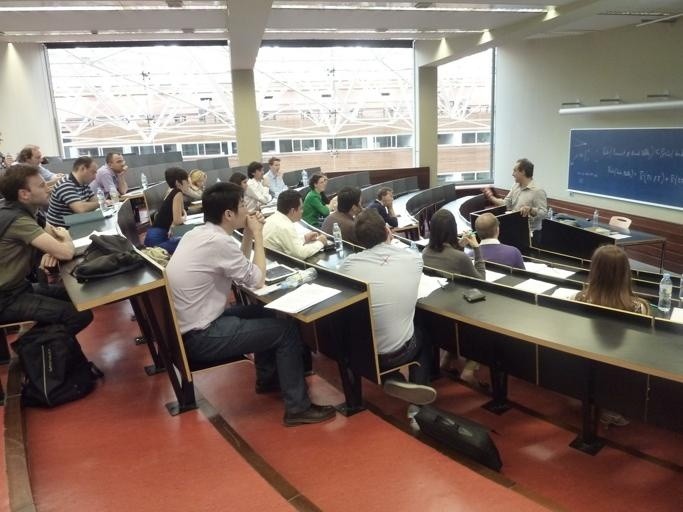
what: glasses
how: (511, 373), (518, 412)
(438, 273), (456, 289)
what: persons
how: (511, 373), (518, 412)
(337, 208), (438, 406)
(562, 244), (650, 317)
(166, 179), (337, 427)
(464, 212), (528, 273)
(230, 156), (399, 262)
(480, 157), (550, 250)
(11, 144), (127, 231)
(144, 166), (208, 254)
(1, 164), (94, 374)
(422, 209), (487, 282)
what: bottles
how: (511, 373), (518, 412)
(279, 266), (318, 291)
(140, 172), (148, 190)
(332, 222), (343, 250)
(409, 241), (418, 251)
(96, 184), (121, 213)
(300, 168), (309, 188)
(592, 211), (598, 225)
(657, 270), (682, 313)
(547, 207), (552, 220)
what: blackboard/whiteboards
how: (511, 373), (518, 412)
(567, 128), (683, 212)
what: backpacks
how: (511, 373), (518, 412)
(13, 322), (105, 411)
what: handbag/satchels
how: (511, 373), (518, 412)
(71, 233), (146, 284)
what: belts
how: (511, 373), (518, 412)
(378, 336), (413, 360)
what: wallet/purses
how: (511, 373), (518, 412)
(462, 288), (486, 302)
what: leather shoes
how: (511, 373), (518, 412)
(283, 402), (337, 427)
(256, 375), (310, 394)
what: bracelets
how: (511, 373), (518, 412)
(528, 206), (532, 213)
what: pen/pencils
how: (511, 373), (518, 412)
(436, 279), (444, 289)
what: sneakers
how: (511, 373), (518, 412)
(599, 410), (631, 427)
(383, 379), (437, 406)
(460, 370), (489, 387)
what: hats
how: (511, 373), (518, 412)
(187, 168), (208, 192)
